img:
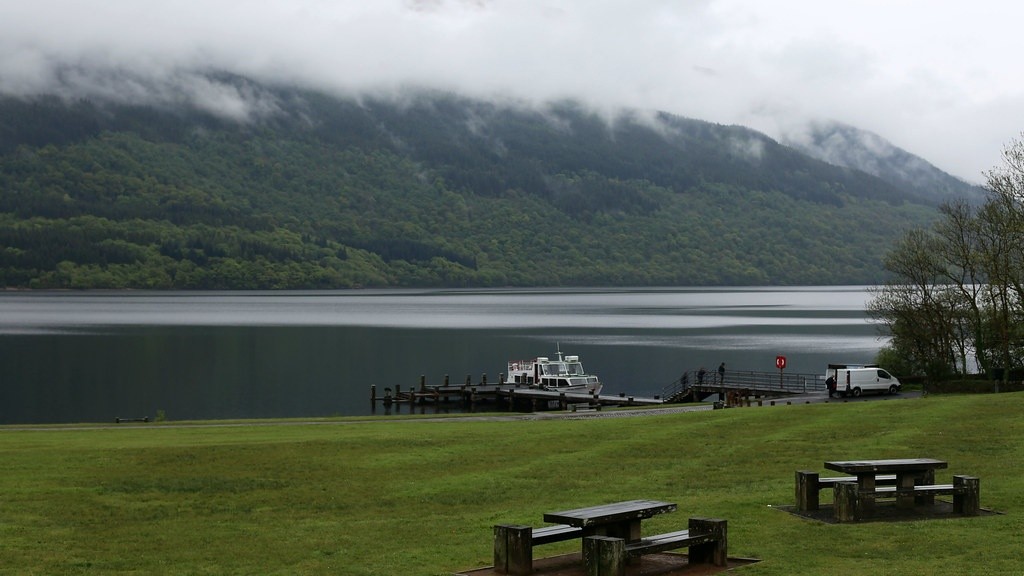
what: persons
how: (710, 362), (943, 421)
(698, 368), (705, 385)
(681, 372), (688, 388)
(718, 362), (725, 383)
(825, 374), (835, 397)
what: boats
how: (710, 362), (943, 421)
(504, 341), (604, 396)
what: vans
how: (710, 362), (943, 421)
(825, 367), (903, 399)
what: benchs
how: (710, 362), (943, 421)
(494, 525), (583, 576)
(833, 474), (980, 522)
(583, 518), (728, 576)
(795, 470), (934, 511)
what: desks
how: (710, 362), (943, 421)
(824, 457), (948, 510)
(544, 499), (678, 565)
